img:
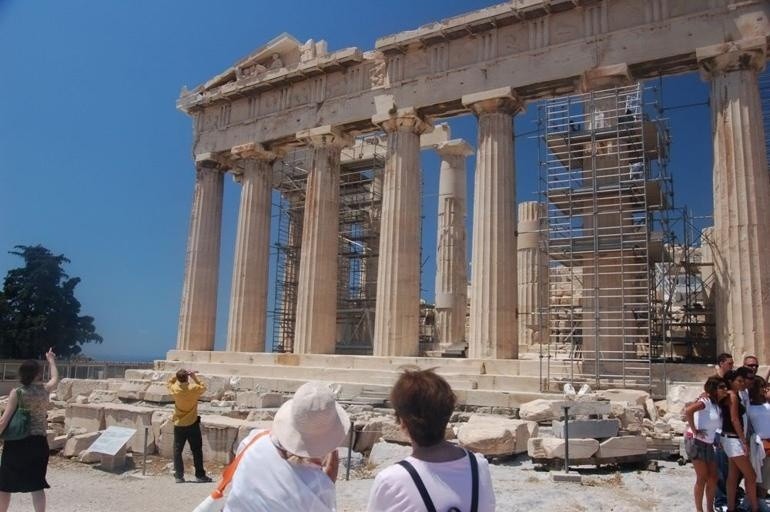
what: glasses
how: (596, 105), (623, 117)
(747, 364), (758, 367)
(718, 385), (725, 389)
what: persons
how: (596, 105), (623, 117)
(166, 369), (212, 483)
(365, 366), (496, 512)
(0, 347), (59, 512)
(191, 380), (350, 512)
(683, 354), (770, 512)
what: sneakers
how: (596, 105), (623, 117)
(196, 476), (212, 482)
(715, 485), (769, 512)
(176, 477), (185, 482)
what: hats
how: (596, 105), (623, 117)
(274, 383), (350, 458)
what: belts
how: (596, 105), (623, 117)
(721, 434), (738, 438)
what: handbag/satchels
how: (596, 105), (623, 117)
(2, 408), (31, 441)
(193, 491), (224, 512)
